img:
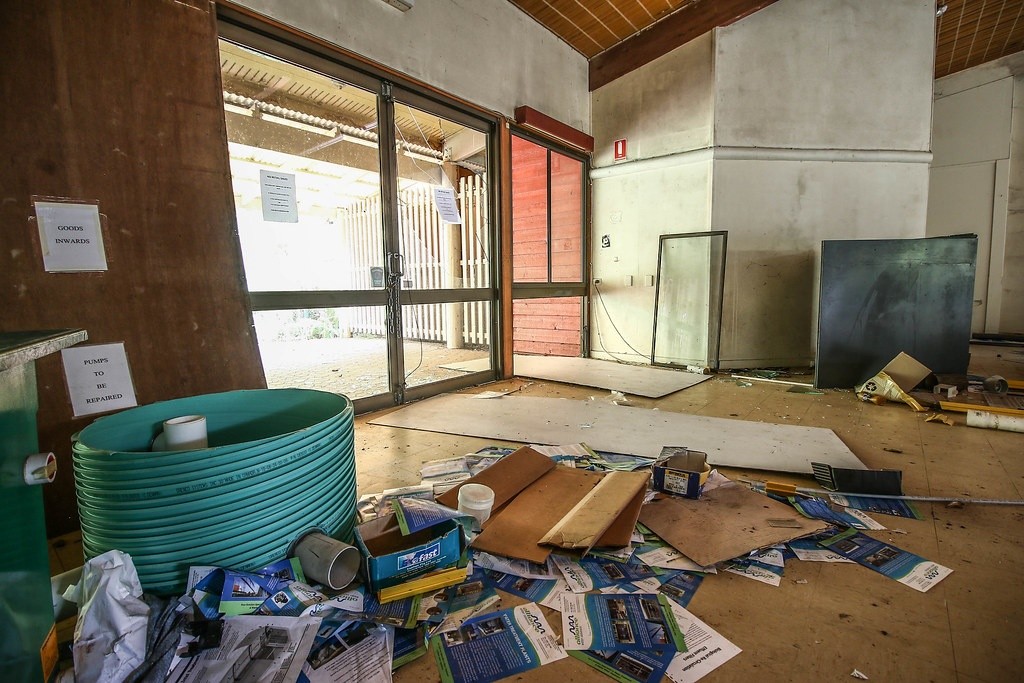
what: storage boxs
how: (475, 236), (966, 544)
(354, 510), (467, 594)
(653, 445), (711, 499)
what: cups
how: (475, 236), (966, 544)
(458, 483), (494, 525)
